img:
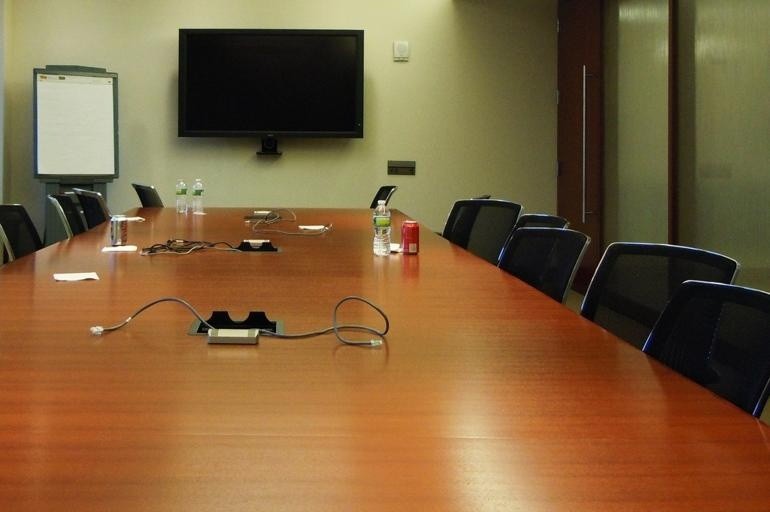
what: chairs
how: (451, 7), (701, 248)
(641, 279), (770, 419)
(370, 186), (400, 209)
(0, 203), (45, 262)
(441, 199), (524, 266)
(47, 193), (85, 238)
(496, 213), (570, 269)
(131, 184), (165, 207)
(497, 226), (592, 305)
(579, 241), (741, 394)
(433, 194), (491, 250)
(73, 188), (110, 229)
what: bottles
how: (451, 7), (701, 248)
(110, 215), (128, 247)
(175, 178), (188, 214)
(191, 178), (205, 215)
(372, 200), (391, 256)
(401, 221), (420, 255)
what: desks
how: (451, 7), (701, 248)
(0, 206), (770, 511)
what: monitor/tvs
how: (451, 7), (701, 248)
(178, 28), (365, 156)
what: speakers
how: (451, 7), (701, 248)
(394, 41), (409, 62)
